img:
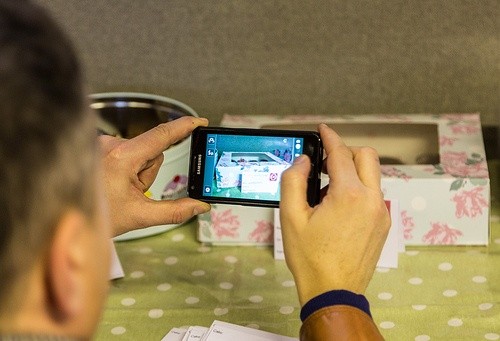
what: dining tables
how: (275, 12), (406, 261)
(94, 160), (499, 341)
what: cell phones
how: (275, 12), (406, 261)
(187, 126), (323, 208)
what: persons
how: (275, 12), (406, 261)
(0, 1), (391, 341)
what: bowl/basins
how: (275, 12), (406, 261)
(86, 92), (199, 241)
